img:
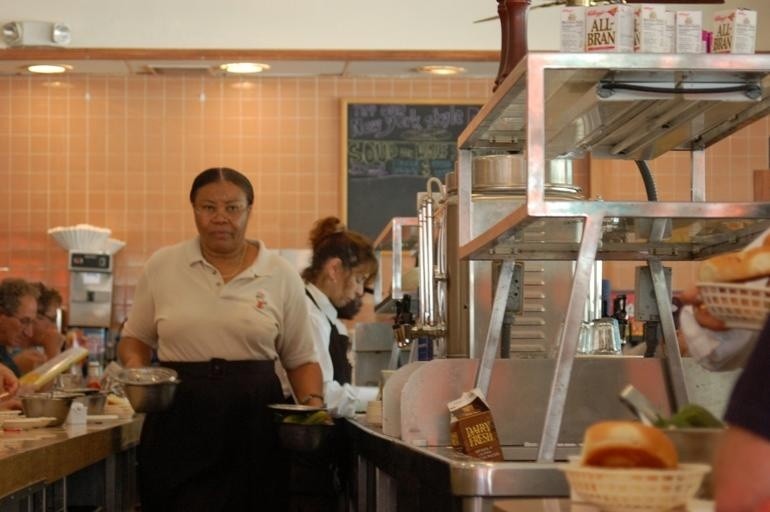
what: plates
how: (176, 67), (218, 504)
(5, 417), (54, 431)
(267, 402), (320, 417)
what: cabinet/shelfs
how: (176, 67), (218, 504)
(373, 217), (419, 312)
(457, 51), (770, 262)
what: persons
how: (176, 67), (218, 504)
(274, 216), (382, 420)
(117, 168), (333, 512)
(0, 278), (89, 411)
(329, 282), (366, 386)
(679, 227), (770, 512)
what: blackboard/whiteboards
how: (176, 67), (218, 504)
(339, 96), (523, 256)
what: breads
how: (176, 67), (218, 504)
(105, 393), (133, 419)
(699, 228), (770, 321)
(582, 419), (677, 470)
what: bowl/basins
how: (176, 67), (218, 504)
(113, 365), (178, 415)
(49, 223), (112, 254)
(654, 423), (729, 501)
(72, 394), (106, 414)
(110, 239), (127, 258)
(22, 396), (74, 427)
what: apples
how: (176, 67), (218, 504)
(87, 382), (100, 389)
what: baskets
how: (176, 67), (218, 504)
(553, 463), (714, 510)
(695, 281), (770, 332)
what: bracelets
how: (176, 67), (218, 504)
(300, 394), (323, 406)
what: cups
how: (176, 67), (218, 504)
(66, 422), (92, 438)
(363, 400), (385, 430)
(68, 401), (87, 425)
(573, 318), (625, 355)
(88, 361), (100, 379)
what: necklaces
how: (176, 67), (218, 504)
(220, 240), (248, 278)
